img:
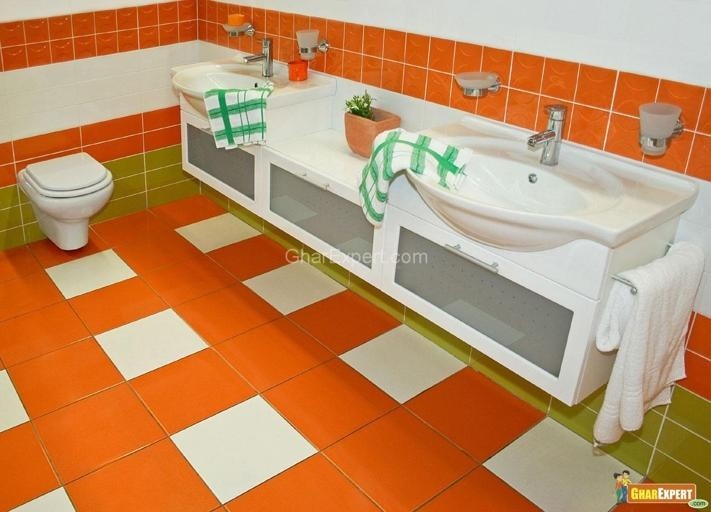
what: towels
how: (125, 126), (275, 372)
(201, 83), (276, 152)
(595, 242), (706, 447)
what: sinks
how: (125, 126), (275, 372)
(404, 123), (700, 250)
(172, 66), (336, 117)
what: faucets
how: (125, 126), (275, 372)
(241, 36), (273, 78)
(525, 103), (569, 167)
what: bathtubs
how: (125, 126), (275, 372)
(0, 39), (252, 252)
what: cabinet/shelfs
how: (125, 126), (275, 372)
(180, 91), (682, 407)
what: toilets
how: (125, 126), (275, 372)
(17, 151), (115, 252)
